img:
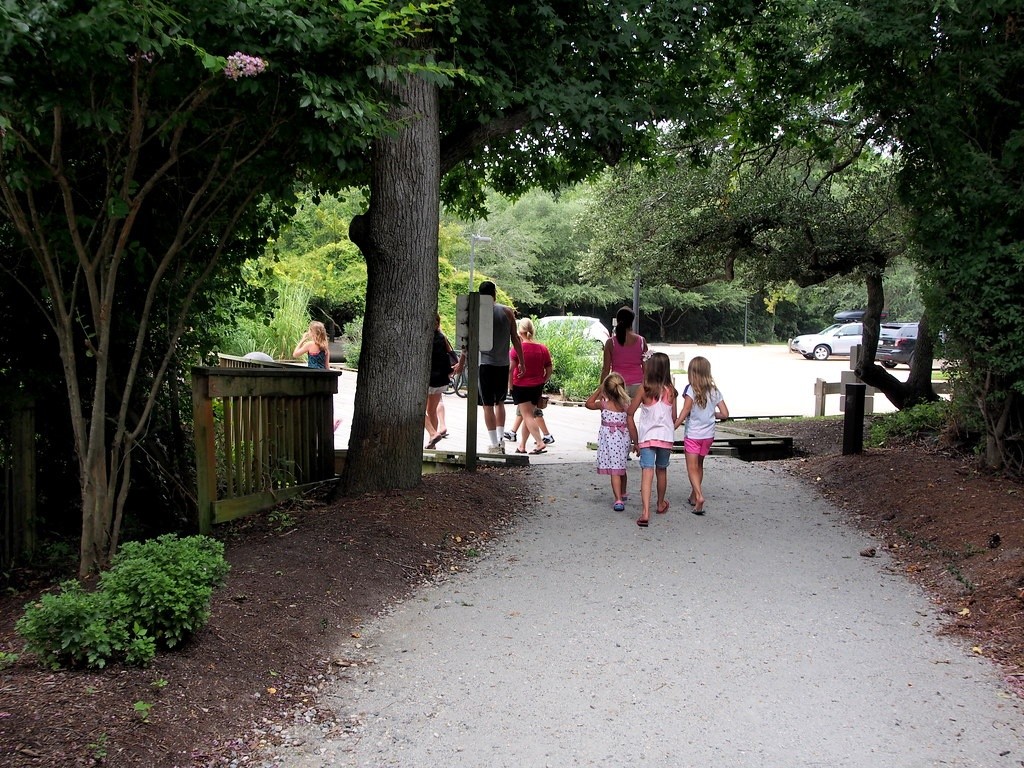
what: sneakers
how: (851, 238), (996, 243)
(534, 435), (555, 444)
(503, 432), (517, 441)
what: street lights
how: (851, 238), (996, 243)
(469, 234), (491, 292)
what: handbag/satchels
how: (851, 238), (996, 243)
(446, 339), (458, 366)
(536, 393), (549, 409)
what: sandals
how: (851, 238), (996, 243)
(614, 500), (624, 511)
(621, 496), (627, 501)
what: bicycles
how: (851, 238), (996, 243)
(442, 348), (469, 398)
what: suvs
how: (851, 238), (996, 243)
(876, 323), (946, 371)
(536, 316), (610, 351)
(790, 311), (889, 360)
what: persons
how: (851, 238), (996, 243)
(509, 317), (552, 455)
(627, 349), (678, 526)
(291, 321), (329, 368)
(599, 306), (648, 461)
(424, 314), (452, 448)
(453, 282), (524, 455)
(504, 398), (555, 445)
(585, 374), (632, 511)
(675, 356), (728, 513)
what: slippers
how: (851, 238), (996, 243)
(425, 434), (442, 449)
(439, 434), (449, 438)
(657, 500), (669, 512)
(691, 506), (705, 513)
(515, 448), (527, 453)
(637, 517), (648, 526)
(687, 496), (696, 506)
(529, 449), (547, 454)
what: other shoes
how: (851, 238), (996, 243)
(489, 438), (505, 454)
